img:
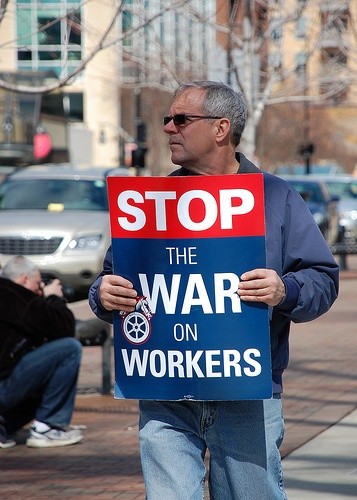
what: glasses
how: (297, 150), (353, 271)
(164, 114), (226, 125)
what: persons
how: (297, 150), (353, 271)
(0, 255), (83, 449)
(88, 85), (339, 500)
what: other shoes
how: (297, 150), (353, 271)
(0, 438), (17, 448)
(26, 426), (83, 447)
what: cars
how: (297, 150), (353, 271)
(0, 161), (138, 304)
(272, 161), (357, 254)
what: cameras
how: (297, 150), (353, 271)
(42, 276), (55, 285)
